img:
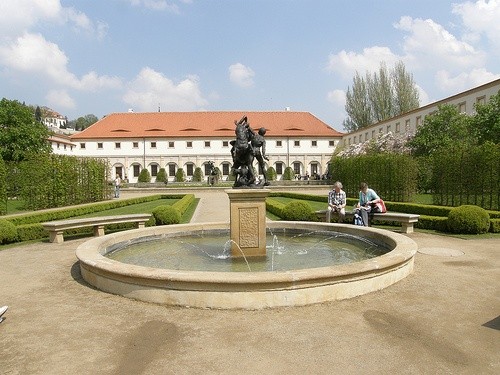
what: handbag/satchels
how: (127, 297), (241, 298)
(370, 200), (386, 213)
(354, 214), (364, 226)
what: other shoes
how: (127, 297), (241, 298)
(0, 317), (3, 323)
(0, 306), (9, 316)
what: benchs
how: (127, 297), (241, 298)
(40, 213), (151, 243)
(315, 210), (420, 234)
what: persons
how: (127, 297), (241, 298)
(114, 174), (121, 198)
(354, 183), (380, 227)
(295, 171), (331, 180)
(124, 176), (129, 183)
(248, 127), (269, 186)
(326, 182), (346, 223)
